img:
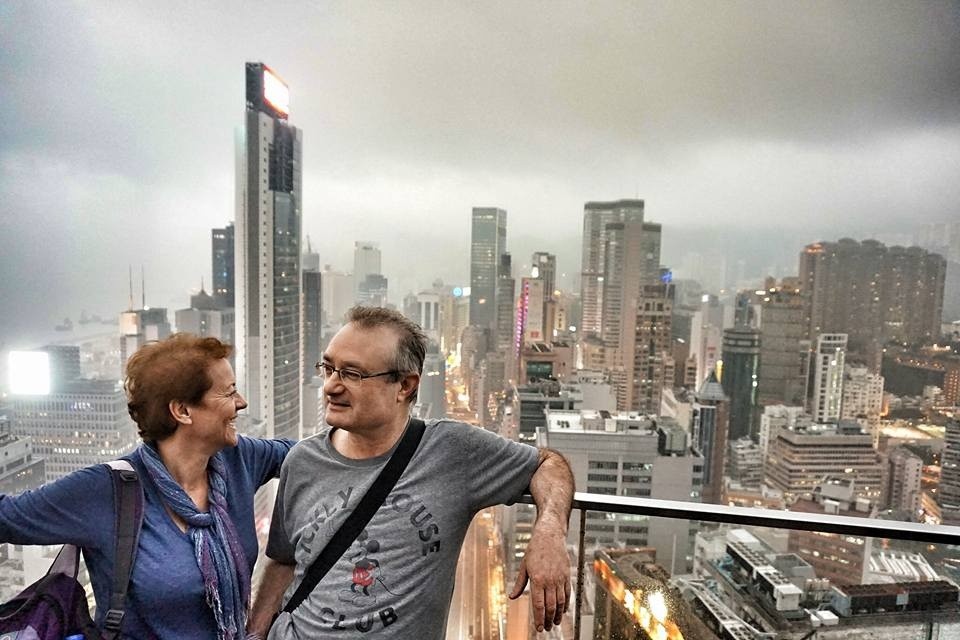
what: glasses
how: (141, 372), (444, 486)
(313, 360), (398, 387)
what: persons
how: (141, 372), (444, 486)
(0, 332), (300, 640)
(248, 304), (575, 640)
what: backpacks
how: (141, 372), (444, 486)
(0, 457), (145, 640)
